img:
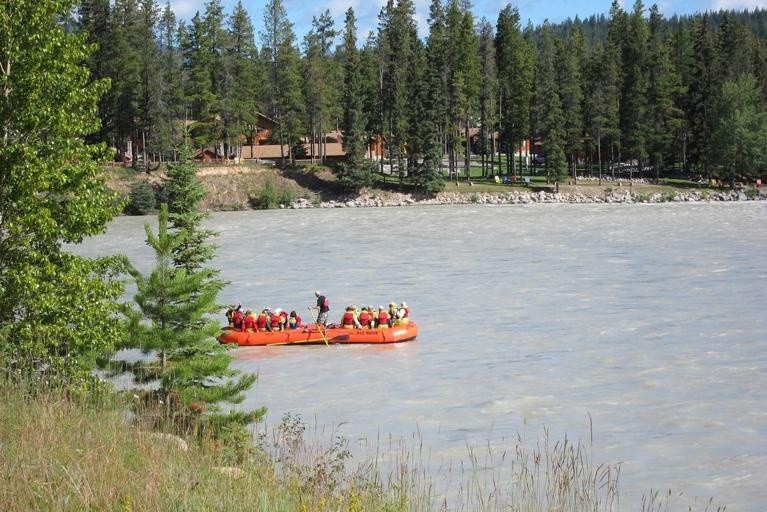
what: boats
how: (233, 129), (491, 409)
(217, 323), (422, 348)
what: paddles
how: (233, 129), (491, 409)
(305, 304), (329, 346)
(267, 335), (349, 346)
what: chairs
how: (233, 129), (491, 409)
(494, 175), (531, 187)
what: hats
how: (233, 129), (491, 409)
(368, 305), (375, 313)
(345, 305), (358, 313)
(379, 302), (406, 310)
(264, 306), (282, 316)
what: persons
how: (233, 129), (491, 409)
(223, 289), (409, 332)
